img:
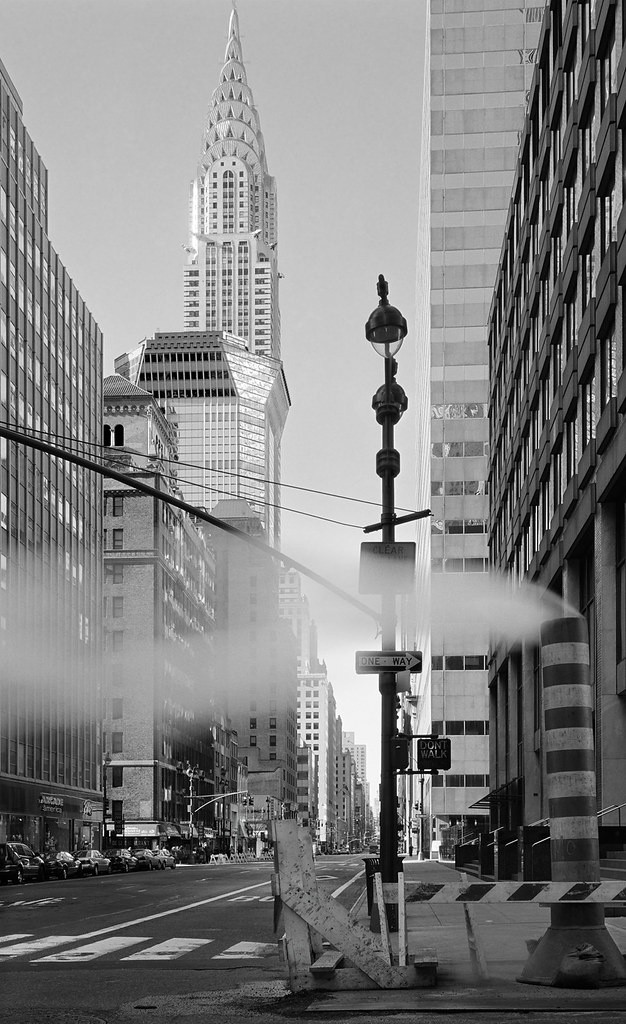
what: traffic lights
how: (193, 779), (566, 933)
(243, 797), (247, 806)
(249, 796), (254, 806)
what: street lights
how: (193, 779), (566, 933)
(364, 271), (408, 938)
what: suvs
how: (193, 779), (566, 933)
(0, 840), (49, 885)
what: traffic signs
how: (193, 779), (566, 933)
(355, 649), (423, 674)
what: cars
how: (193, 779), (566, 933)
(129, 848), (162, 871)
(103, 848), (139, 874)
(151, 848), (177, 870)
(71, 850), (113, 876)
(36, 850), (83, 880)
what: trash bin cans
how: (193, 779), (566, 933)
(361, 856), (407, 917)
